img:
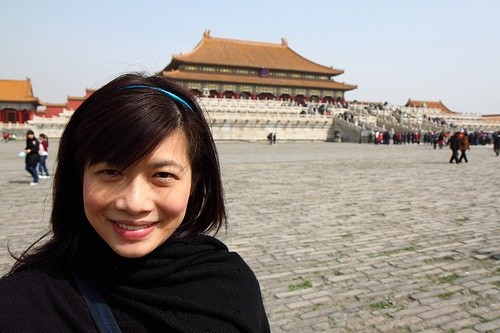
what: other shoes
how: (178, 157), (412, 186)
(38, 174), (50, 180)
(30, 182), (38, 185)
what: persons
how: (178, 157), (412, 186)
(369, 128), (500, 164)
(0, 73), (271, 333)
(25, 130), (50, 185)
(267, 133), (276, 145)
